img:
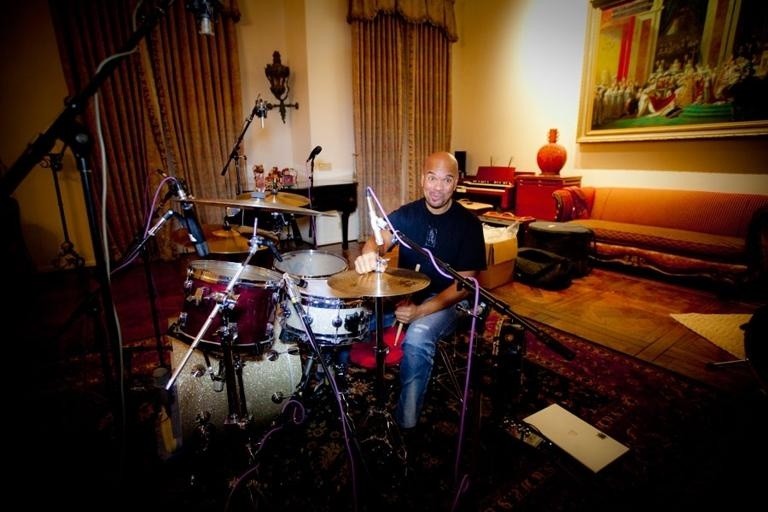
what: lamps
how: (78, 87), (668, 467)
(264, 51), (298, 123)
(199, 10), (215, 36)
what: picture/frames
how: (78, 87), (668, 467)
(576, 1), (768, 143)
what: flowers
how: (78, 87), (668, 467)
(265, 167), (285, 190)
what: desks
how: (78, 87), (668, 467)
(242, 176), (360, 250)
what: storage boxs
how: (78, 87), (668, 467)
(514, 175), (582, 221)
(475, 223), (518, 289)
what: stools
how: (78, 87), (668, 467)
(396, 316), (476, 413)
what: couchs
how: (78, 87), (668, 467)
(551, 186), (768, 304)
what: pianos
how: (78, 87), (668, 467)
(227, 179), (358, 250)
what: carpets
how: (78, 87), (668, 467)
(65, 309), (723, 512)
(668, 313), (754, 360)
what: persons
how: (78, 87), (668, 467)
(352, 151), (488, 449)
(592, 33), (768, 125)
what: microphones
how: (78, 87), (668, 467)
(306, 145), (322, 162)
(366, 188), (385, 246)
(171, 179), (210, 257)
(283, 272), (308, 289)
(258, 98), (266, 128)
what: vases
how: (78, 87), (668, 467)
(537, 129), (567, 175)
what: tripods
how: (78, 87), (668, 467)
(326, 298), (416, 511)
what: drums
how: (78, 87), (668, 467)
(271, 250), (350, 311)
(176, 259), (283, 350)
(280, 275), (368, 349)
(166, 318), (303, 435)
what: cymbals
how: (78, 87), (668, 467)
(235, 191), (311, 207)
(327, 267), (430, 301)
(170, 224), (279, 255)
(172, 197), (338, 218)
